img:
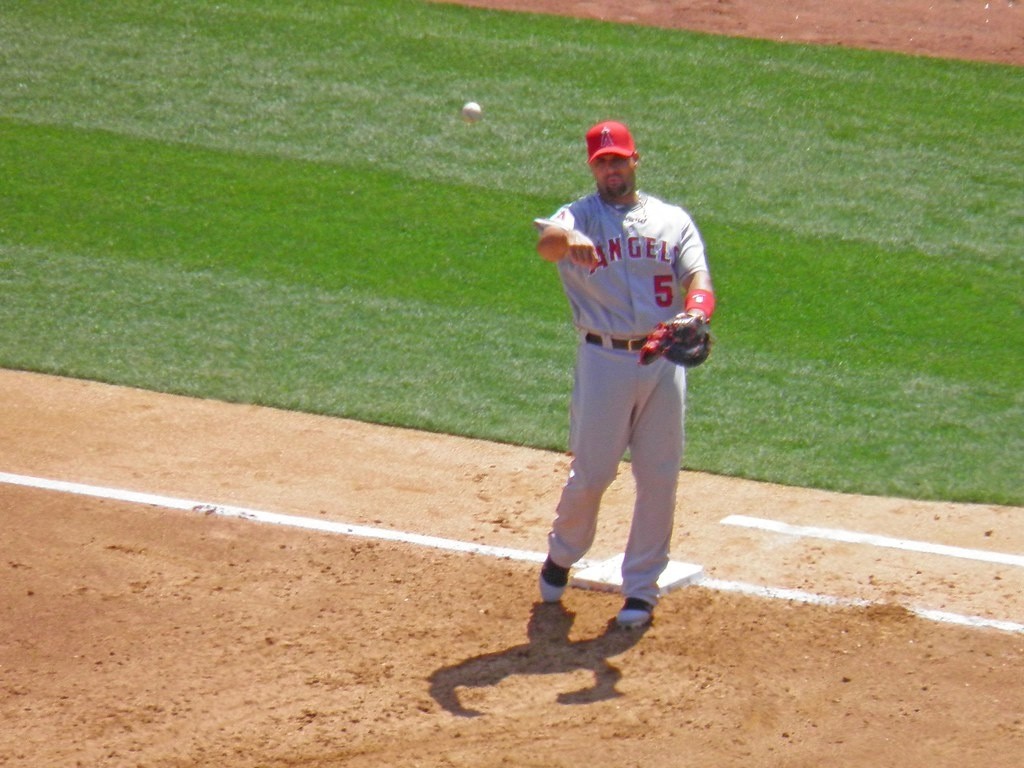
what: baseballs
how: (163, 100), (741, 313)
(462, 102), (481, 121)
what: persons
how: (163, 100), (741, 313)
(534, 120), (714, 628)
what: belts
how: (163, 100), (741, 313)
(586, 333), (647, 352)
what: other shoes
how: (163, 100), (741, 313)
(616, 597), (654, 627)
(538, 554), (569, 603)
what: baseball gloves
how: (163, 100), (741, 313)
(633, 313), (713, 368)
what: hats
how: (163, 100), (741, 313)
(585, 121), (635, 164)
